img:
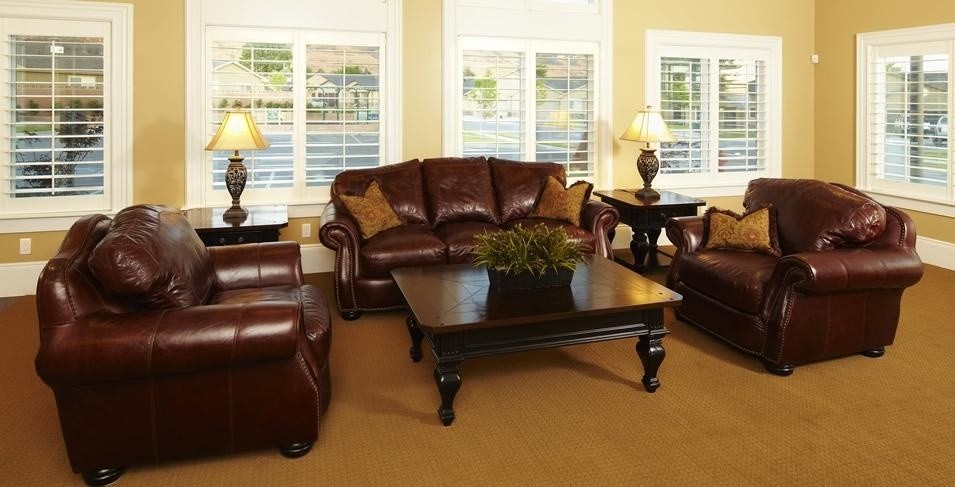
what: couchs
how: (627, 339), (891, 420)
(664, 177), (925, 377)
(33, 212), (333, 486)
(314, 156), (622, 321)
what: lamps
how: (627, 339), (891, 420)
(203, 110), (272, 218)
(618, 109), (679, 200)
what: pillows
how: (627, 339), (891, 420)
(525, 175), (595, 227)
(334, 177), (406, 241)
(703, 204), (782, 257)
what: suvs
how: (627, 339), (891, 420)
(933, 116), (947, 145)
(894, 109), (948, 141)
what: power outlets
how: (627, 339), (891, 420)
(18, 238), (32, 256)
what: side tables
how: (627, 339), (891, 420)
(592, 188), (707, 273)
(174, 200), (290, 247)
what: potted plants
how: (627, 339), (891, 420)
(467, 223), (597, 294)
